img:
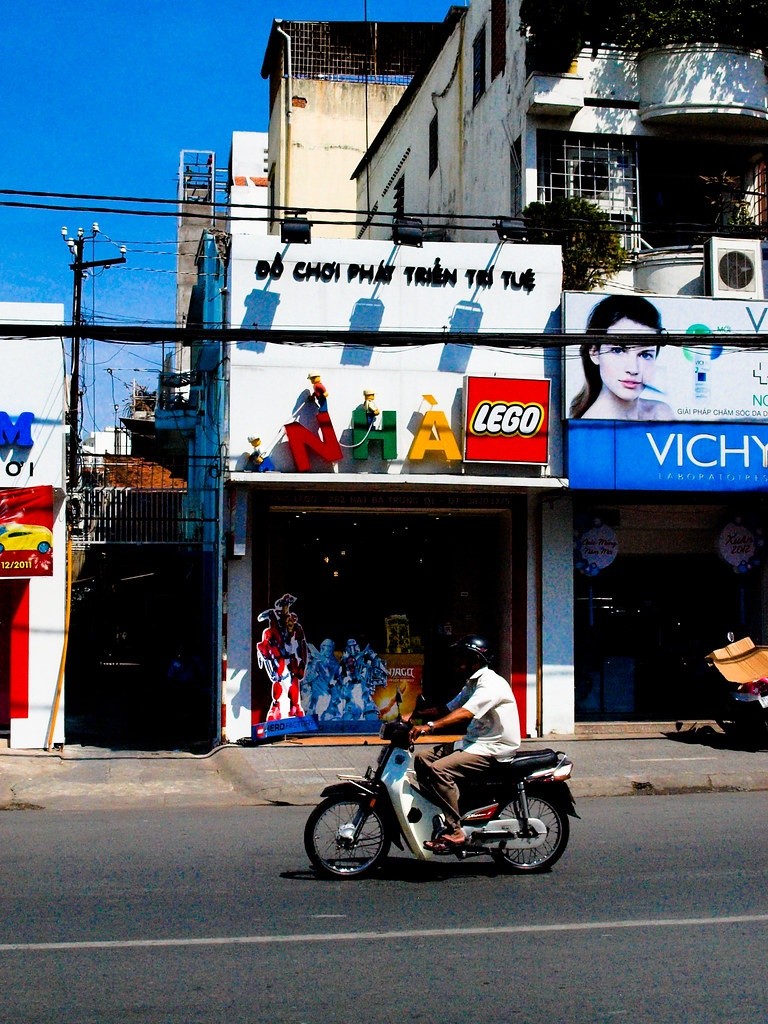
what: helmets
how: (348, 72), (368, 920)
(450, 634), (493, 663)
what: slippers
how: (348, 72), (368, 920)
(423, 836), (466, 851)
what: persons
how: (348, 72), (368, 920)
(244, 435), (275, 472)
(409, 634), (522, 851)
(569, 296), (674, 420)
(363, 390), (380, 431)
(307, 373), (328, 412)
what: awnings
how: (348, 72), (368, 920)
(563, 418), (768, 493)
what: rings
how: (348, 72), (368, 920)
(410, 731), (413, 733)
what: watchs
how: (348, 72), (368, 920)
(427, 722), (433, 731)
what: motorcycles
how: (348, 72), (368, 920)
(304, 690), (582, 880)
(726, 632), (768, 744)
